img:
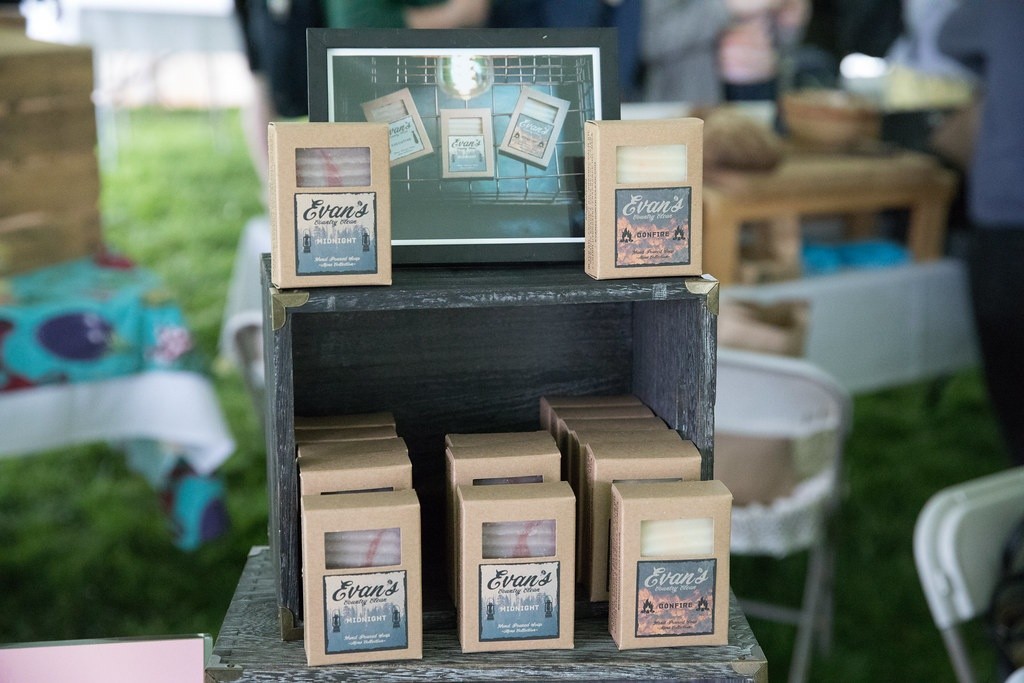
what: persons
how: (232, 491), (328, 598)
(938, 0), (1024, 468)
(230, 0), (932, 210)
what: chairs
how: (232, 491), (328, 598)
(715, 349), (856, 683)
(910, 469), (1024, 683)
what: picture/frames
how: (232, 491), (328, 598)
(306, 26), (621, 265)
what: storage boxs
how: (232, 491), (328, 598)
(266, 121), (393, 289)
(583, 113), (703, 281)
(608, 480), (733, 652)
(260, 252), (720, 643)
(444, 437), (561, 611)
(203, 546), (769, 683)
(295, 412), (413, 495)
(299, 489), (423, 667)
(456, 480), (575, 654)
(445, 430), (556, 448)
(538, 395), (703, 603)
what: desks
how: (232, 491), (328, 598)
(702, 144), (957, 287)
(0, 253), (238, 555)
(209, 218), (977, 394)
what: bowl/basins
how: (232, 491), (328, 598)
(781, 88), (879, 150)
